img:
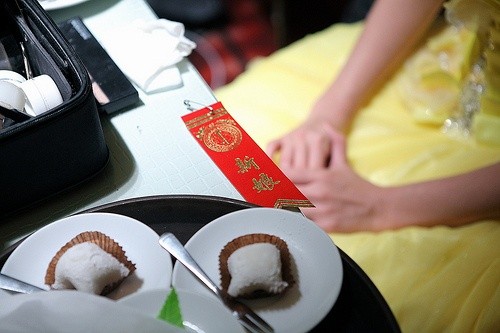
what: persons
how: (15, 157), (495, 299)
(213, 0), (500, 333)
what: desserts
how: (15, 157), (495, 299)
(225, 242), (288, 296)
(50, 241), (130, 295)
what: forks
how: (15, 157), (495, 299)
(158, 233), (274, 333)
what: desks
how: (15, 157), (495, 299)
(1, 0), (300, 258)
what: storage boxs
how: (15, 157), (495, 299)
(0, 0), (109, 221)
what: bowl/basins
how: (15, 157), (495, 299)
(0, 292), (205, 333)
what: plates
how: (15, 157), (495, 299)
(172, 208), (342, 333)
(0, 213), (173, 306)
